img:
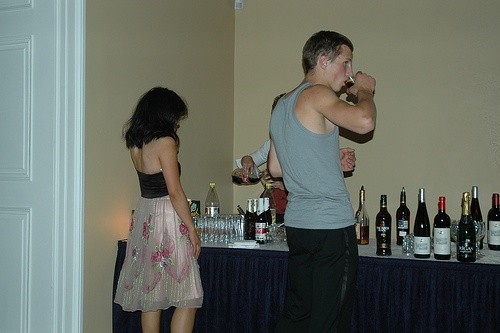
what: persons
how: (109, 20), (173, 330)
(235, 31), (377, 333)
(114, 87), (204, 332)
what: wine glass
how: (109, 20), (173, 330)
(451, 220), (485, 259)
(192, 214), (243, 243)
(402, 233), (414, 256)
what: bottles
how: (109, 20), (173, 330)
(353, 185), (370, 245)
(243, 198), (273, 244)
(230, 167), (264, 186)
(205, 183), (220, 218)
(262, 183), (277, 225)
(456, 192), (477, 262)
(374, 195), (392, 256)
(471, 186), (485, 249)
(487, 193), (500, 251)
(414, 188), (431, 258)
(433, 196), (451, 261)
(395, 187), (410, 246)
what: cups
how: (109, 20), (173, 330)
(344, 73), (375, 97)
(259, 169), (274, 189)
(268, 223), (287, 245)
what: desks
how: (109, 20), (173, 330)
(112, 231), (500, 333)
